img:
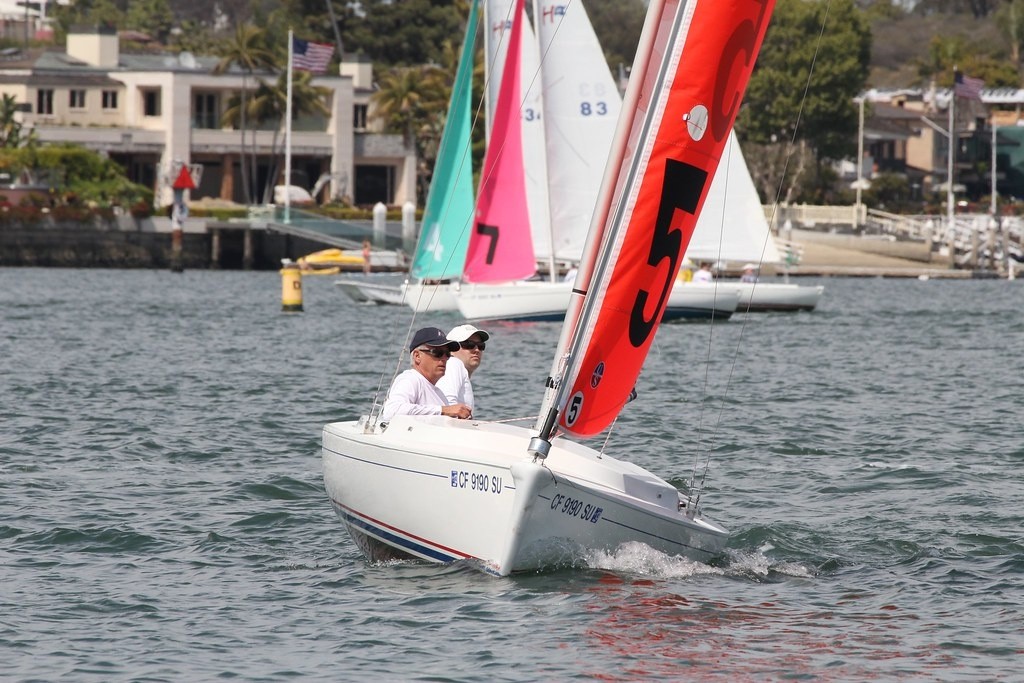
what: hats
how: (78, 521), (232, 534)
(742, 264), (761, 270)
(446, 324), (490, 342)
(409, 327), (460, 353)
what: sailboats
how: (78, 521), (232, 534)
(201, 0), (825, 326)
(316, 0), (776, 582)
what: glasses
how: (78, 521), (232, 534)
(459, 340), (485, 351)
(420, 349), (451, 359)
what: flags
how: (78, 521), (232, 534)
(293, 35), (334, 74)
(954, 70), (986, 99)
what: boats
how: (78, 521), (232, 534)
(296, 241), (407, 275)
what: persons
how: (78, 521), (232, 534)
(434, 324), (490, 420)
(741, 269), (759, 282)
(863, 149), (874, 178)
(361, 239), (371, 272)
(692, 262), (714, 280)
(382, 327), (472, 419)
